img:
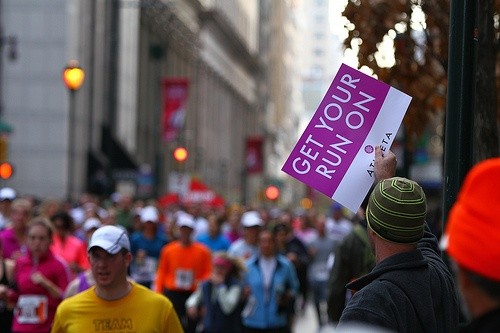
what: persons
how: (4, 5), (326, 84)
(335, 145), (462, 333)
(51, 225), (184, 333)
(0, 183), (366, 333)
(441, 158), (500, 333)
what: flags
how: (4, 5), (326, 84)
(244, 137), (264, 174)
(161, 78), (190, 141)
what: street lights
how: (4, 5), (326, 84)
(62, 59), (84, 203)
(175, 148), (188, 199)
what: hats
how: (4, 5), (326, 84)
(139, 206), (159, 223)
(0, 188), (15, 200)
(241, 211), (265, 226)
(365, 177), (426, 242)
(446, 158), (500, 282)
(177, 214), (194, 228)
(83, 219), (102, 232)
(87, 225), (129, 254)
(68, 209), (85, 225)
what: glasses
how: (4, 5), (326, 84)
(88, 252), (120, 264)
